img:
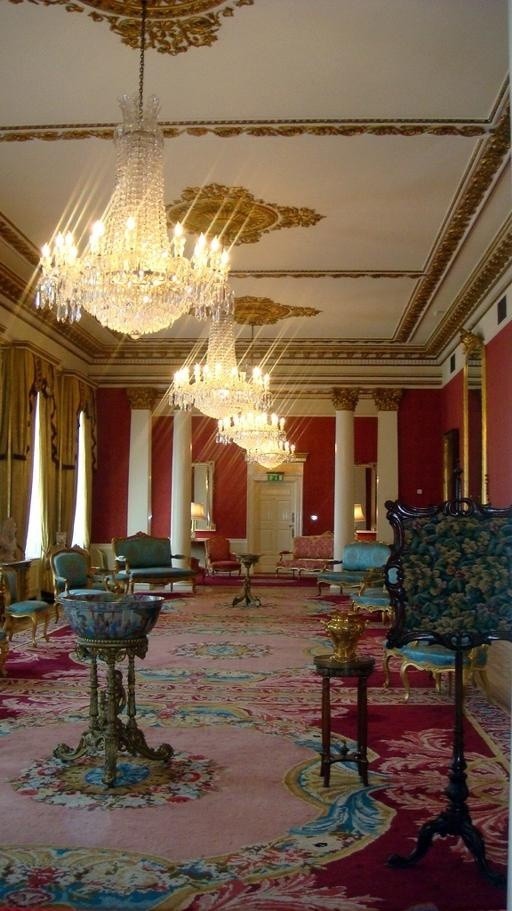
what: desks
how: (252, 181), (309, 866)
(231, 551), (264, 606)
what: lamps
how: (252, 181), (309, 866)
(352, 504), (366, 528)
(34, 0), (231, 337)
(190, 502), (205, 531)
(171, 231), (295, 470)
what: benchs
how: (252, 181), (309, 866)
(315, 543), (393, 595)
(274, 534), (334, 580)
(111, 531), (201, 597)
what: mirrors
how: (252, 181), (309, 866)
(192, 462), (217, 531)
(352, 464), (376, 530)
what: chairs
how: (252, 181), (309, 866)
(383, 636), (492, 698)
(49, 544), (120, 624)
(204, 536), (242, 577)
(2, 574), (49, 646)
(352, 564), (396, 624)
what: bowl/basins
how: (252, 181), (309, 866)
(55, 590), (166, 639)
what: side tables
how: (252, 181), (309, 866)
(55, 635), (174, 784)
(313, 654), (376, 786)
